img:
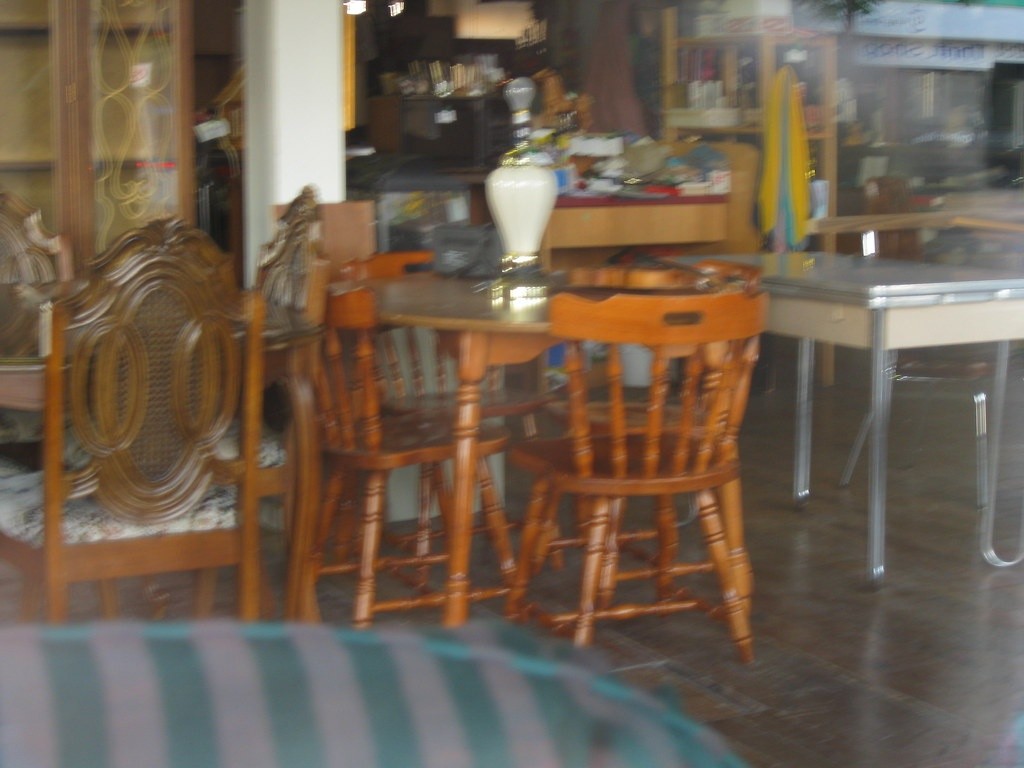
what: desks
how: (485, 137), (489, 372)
(665, 251), (1024, 582)
(539, 194), (732, 389)
(373, 275), (710, 640)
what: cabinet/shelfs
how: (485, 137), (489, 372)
(661, 6), (837, 389)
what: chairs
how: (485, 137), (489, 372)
(0, 185), (1024, 668)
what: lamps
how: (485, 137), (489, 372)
(484, 77), (561, 299)
